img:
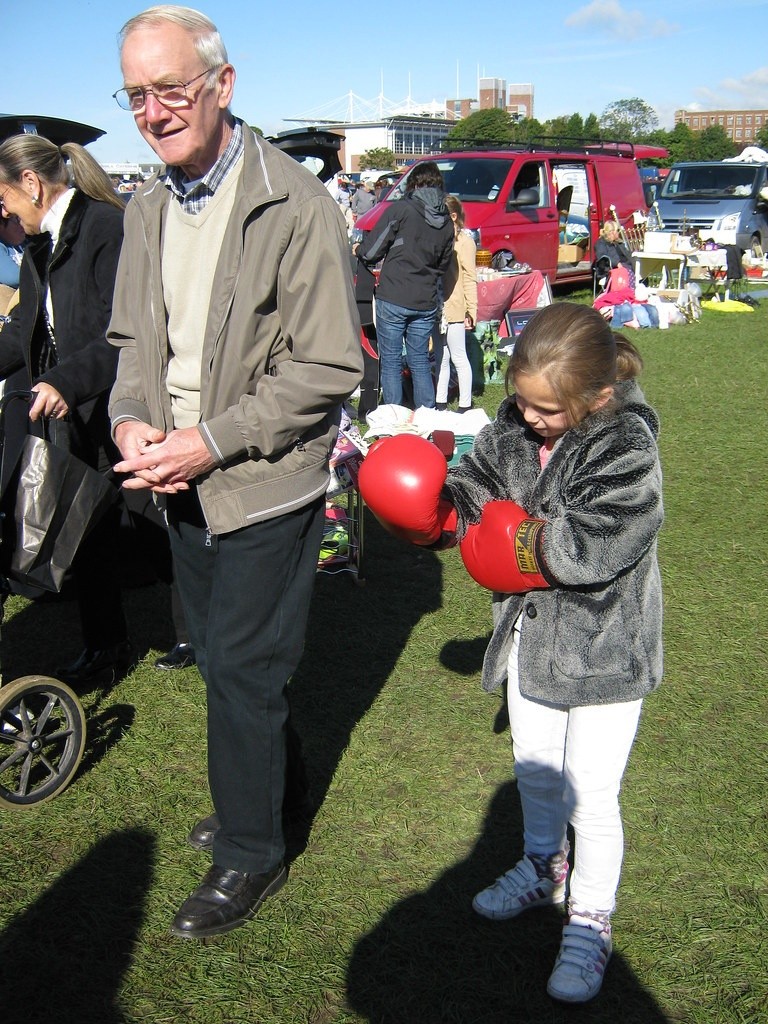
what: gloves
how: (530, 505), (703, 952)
(460, 500), (557, 594)
(358, 435), (458, 552)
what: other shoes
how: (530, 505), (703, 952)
(456, 403), (475, 414)
(436, 403), (447, 412)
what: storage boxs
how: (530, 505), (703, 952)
(558, 243), (585, 262)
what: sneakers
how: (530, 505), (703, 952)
(548, 914), (613, 1002)
(472, 856), (568, 920)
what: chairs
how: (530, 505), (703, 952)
(592, 257), (615, 300)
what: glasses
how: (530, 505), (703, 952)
(112, 68), (212, 111)
(0, 179), (18, 204)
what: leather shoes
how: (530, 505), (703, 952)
(189, 812), (222, 850)
(173, 864), (289, 936)
(154, 643), (198, 670)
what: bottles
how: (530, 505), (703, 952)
(476, 250), (492, 269)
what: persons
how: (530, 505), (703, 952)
(336, 180), (393, 237)
(360, 300), (664, 1003)
(352, 161), (478, 413)
(594, 220), (649, 287)
(0, 2), (364, 937)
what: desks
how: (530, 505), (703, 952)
(632, 250), (696, 285)
(685, 249), (754, 300)
(471, 269), (551, 338)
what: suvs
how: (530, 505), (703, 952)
(266, 127), (344, 184)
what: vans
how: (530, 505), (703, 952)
(376, 167), (454, 189)
(346, 135), (670, 286)
(646, 159), (768, 255)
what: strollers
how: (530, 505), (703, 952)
(0, 392), (89, 813)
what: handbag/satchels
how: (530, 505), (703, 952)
(2, 414), (110, 595)
